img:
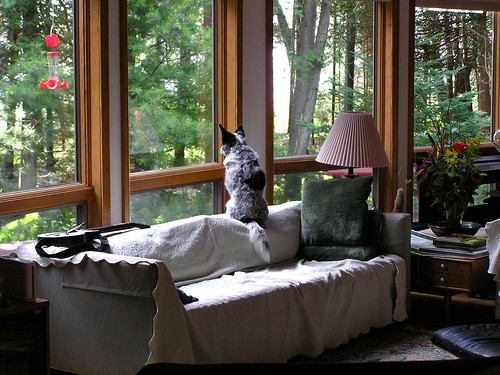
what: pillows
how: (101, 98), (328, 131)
(301, 177), (384, 246)
(301, 244), (382, 262)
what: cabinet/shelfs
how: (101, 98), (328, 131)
(409, 228), (496, 328)
(0, 297), (50, 375)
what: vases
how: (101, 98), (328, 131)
(428, 201), (482, 236)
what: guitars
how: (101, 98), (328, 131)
(36, 223), (150, 247)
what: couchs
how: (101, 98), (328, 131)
(1, 200), (412, 375)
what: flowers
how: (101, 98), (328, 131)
(419, 109), (485, 214)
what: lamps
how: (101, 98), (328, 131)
(315, 111), (390, 178)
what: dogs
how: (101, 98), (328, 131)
(218, 123), (271, 263)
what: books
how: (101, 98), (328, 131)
(433, 233), (487, 251)
